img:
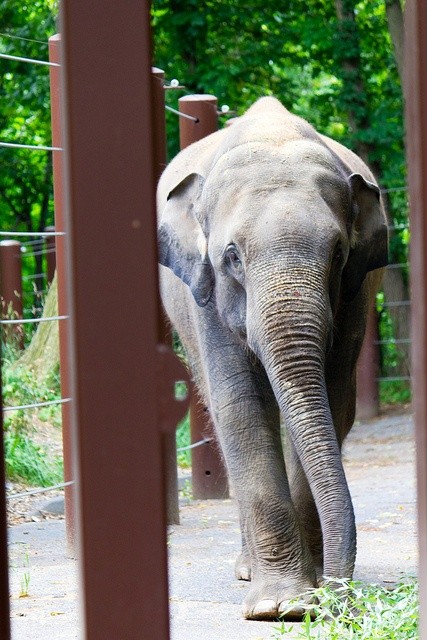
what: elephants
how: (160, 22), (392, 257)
(156, 96), (388, 619)
